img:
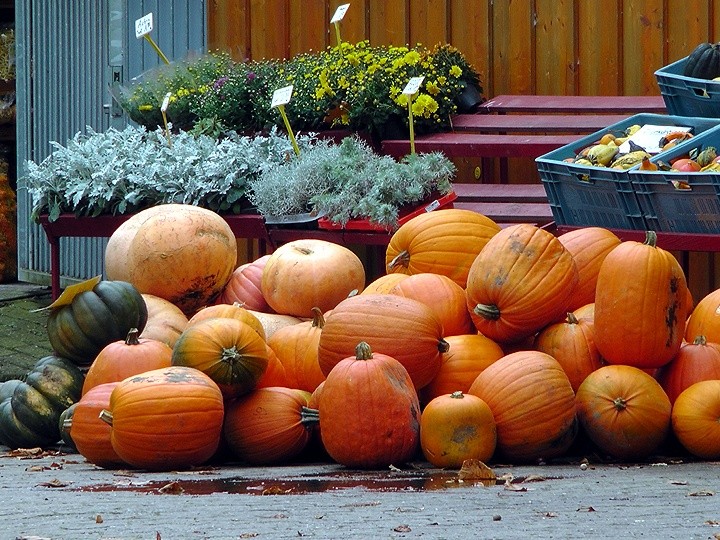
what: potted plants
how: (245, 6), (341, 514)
(15, 114), (460, 237)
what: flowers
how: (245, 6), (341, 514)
(108, 30), (484, 153)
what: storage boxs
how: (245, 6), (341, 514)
(532, 40), (720, 236)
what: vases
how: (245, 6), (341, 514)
(370, 111), (412, 156)
(445, 75), (485, 114)
(412, 96), (452, 137)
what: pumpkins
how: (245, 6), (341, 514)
(683, 41), (720, 82)
(0, 204), (719, 469)
(563, 123), (720, 189)
